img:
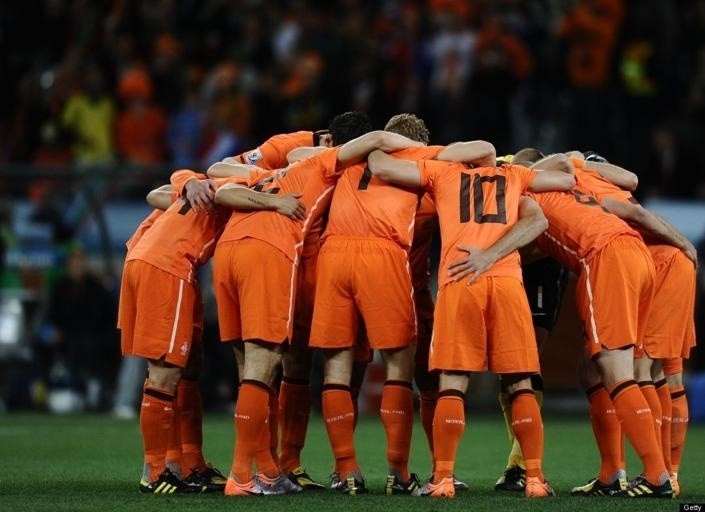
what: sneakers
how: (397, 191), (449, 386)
(494, 467), (526, 491)
(225, 472), (287, 495)
(255, 472), (304, 495)
(329, 471), (366, 496)
(429, 475), (468, 490)
(384, 473), (421, 496)
(613, 472), (681, 499)
(525, 476), (556, 497)
(138, 463), (228, 497)
(411, 477), (455, 497)
(288, 466), (326, 491)
(570, 476), (629, 497)
